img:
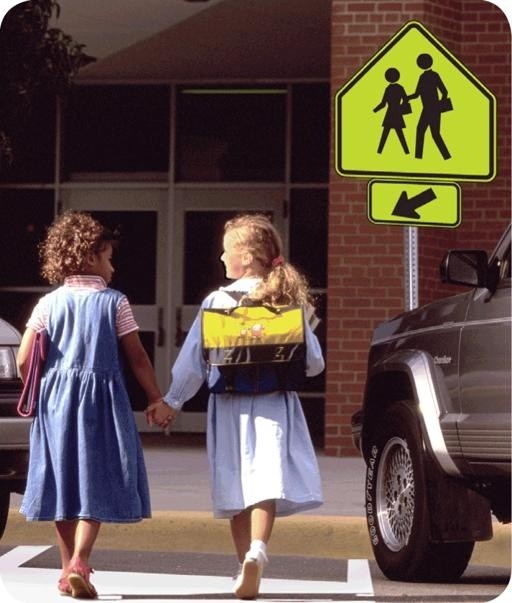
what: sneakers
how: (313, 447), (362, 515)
(232, 553), (262, 599)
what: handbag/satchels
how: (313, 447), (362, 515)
(16, 329), (48, 418)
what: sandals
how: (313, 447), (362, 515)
(58, 561), (98, 599)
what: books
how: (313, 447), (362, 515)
(305, 302), (316, 320)
(309, 314), (321, 332)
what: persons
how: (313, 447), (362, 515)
(142, 212), (330, 600)
(14, 209), (174, 601)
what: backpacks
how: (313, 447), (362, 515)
(199, 289), (309, 397)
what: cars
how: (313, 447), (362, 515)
(350, 222), (512, 581)
